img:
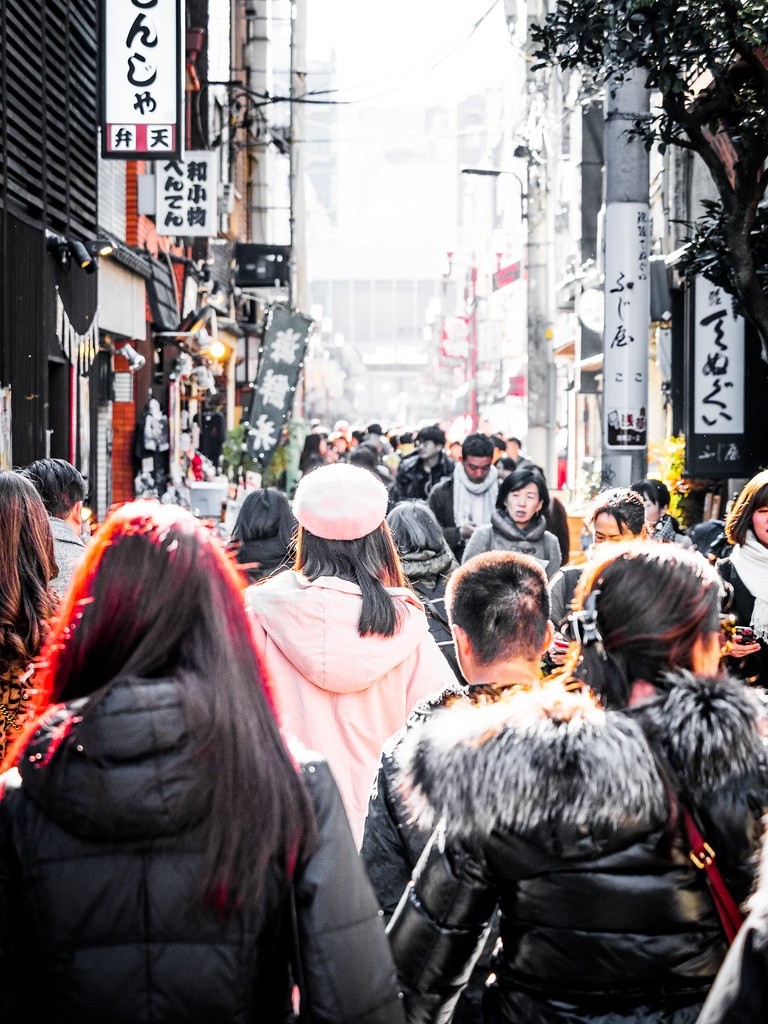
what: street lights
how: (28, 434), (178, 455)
(457, 164), (553, 484)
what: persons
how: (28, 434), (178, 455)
(21, 458), (96, 611)
(387, 537), (768, 1024)
(0, 502), (400, 1024)
(719, 469), (768, 691)
(238, 463), (462, 860)
(0, 468), (64, 780)
(360, 551), (608, 928)
(228, 421), (734, 687)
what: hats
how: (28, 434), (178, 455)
(292, 463), (388, 540)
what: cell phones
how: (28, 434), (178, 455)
(734, 626), (754, 645)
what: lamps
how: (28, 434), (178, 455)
(48, 232), (114, 273)
(99, 343), (146, 372)
